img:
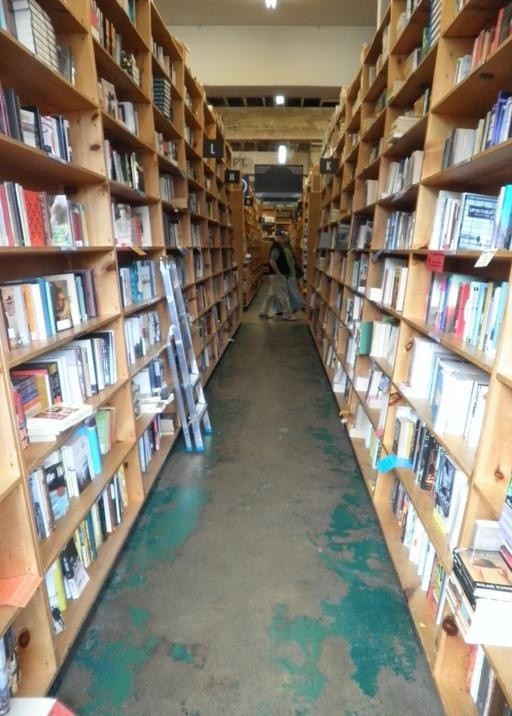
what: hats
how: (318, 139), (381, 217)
(275, 229), (288, 237)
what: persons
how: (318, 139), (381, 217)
(258, 228), (298, 320)
(275, 230), (307, 316)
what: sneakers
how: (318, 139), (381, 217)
(283, 314), (298, 321)
(258, 311), (273, 319)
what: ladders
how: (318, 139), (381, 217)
(160, 254), (213, 453)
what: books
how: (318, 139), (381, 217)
(0, 0), (265, 699)
(291, 0), (512, 715)
